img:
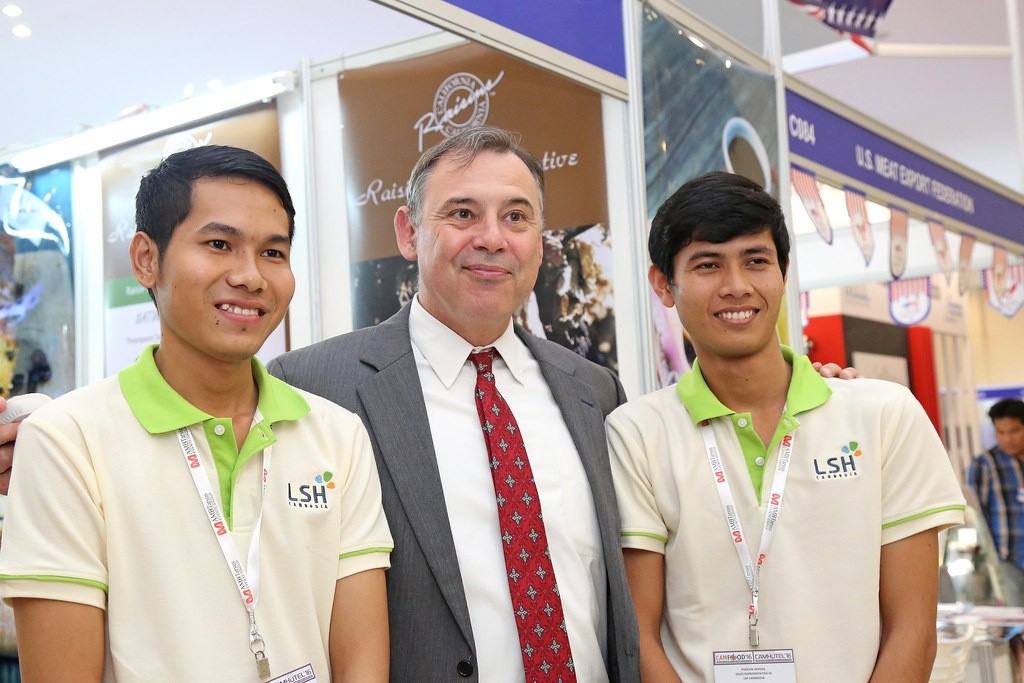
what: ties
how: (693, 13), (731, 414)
(466, 347), (575, 682)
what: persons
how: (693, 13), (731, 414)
(968, 398), (1024, 679)
(0, 145), (394, 683)
(602, 171), (968, 683)
(0, 126), (864, 683)
